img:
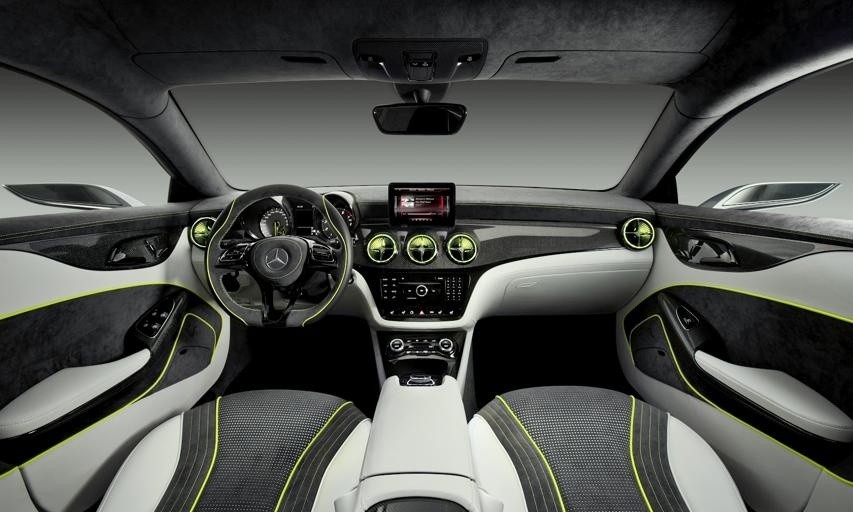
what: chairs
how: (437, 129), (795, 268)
(93, 389), (371, 512)
(467, 386), (751, 511)
